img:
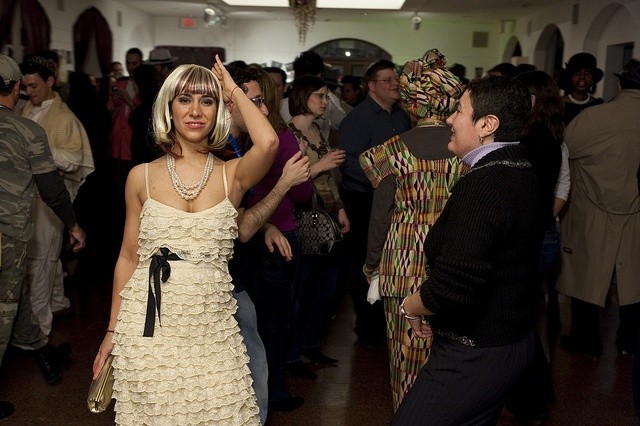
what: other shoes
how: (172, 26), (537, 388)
(36, 343), (74, 386)
(269, 391), (305, 412)
(0, 401), (14, 419)
(546, 302), (561, 334)
(302, 348), (339, 364)
(289, 360), (320, 380)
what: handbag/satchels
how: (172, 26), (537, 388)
(87, 355), (114, 414)
(297, 209), (342, 254)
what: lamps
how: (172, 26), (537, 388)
(412, 11), (422, 31)
(204, 3), (229, 28)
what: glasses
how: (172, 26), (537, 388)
(249, 98), (265, 107)
(311, 93), (329, 100)
(373, 77), (400, 83)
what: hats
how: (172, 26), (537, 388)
(557, 52), (604, 92)
(613, 58), (640, 83)
(0, 55), (23, 83)
(142, 48), (179, 65)
(26, 56), (56, 74)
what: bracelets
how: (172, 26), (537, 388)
(401, 296), (418, 319)
(231, 85), (238, 99)
(106, 329), (114, 332)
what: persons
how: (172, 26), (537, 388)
(358, 48), (470, 413)
(265, 52), (364, 152)
(389, 74), (549, 426)
(209, 67), (315, 426)
(561, 58), (640, 357)
(557, 52), (605, 125)
(72, 47), (179, 164)
(0, 54), (87, 421)
(92, 54), (280, 426)
(449, 63), (537, 87)
(501, 69), (571, 426)
(11, 49), (96, 336)
(285, 73), (350, 380)
(336, 60), (413, 349)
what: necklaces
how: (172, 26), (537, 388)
(167, 152), (214, 200)
(568, 93), (590, 104)
